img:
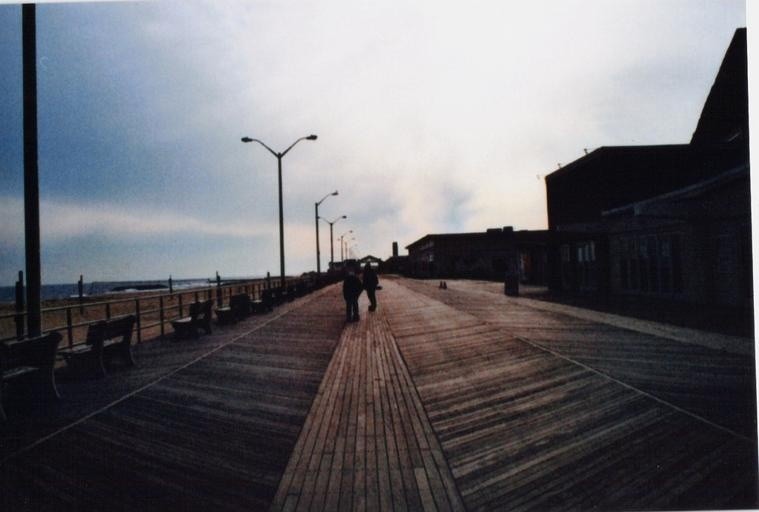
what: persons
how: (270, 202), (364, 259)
(362, 264), (378, 312)
(341, 266), (362, 323)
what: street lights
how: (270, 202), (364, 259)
(315, 190), (358, 274)
(241, 134), (317, 292)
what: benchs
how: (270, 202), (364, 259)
(215, 293), (249, 325)
(0, 329), (64, 421)
(251, 289), (272, 313)
(65, 315), (136, 378)
(170, 300), (214, 339)
